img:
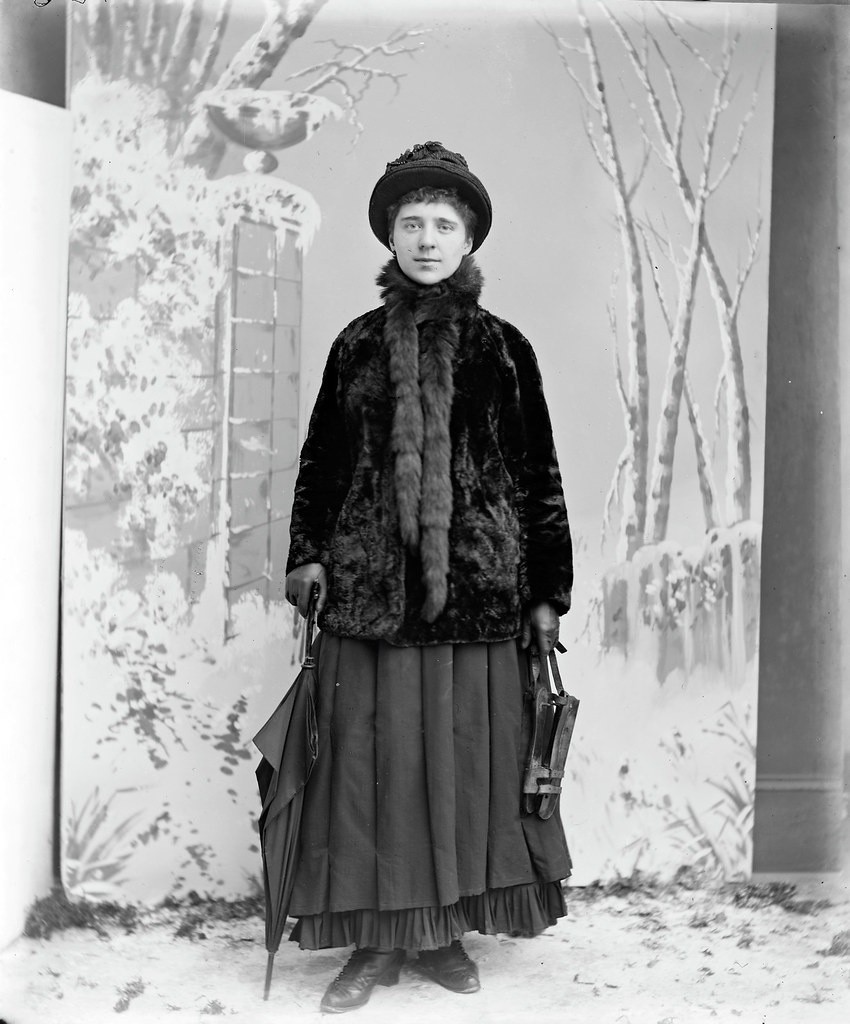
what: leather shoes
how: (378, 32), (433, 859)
(320, 947), (405, 1014)
(419, 939), (480, 993)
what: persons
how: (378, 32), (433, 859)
(265, 141), (573, 1012)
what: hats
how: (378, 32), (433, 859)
(368, 142), (492, 256)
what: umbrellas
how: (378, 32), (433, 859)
(250, 584), (320, 1000)
